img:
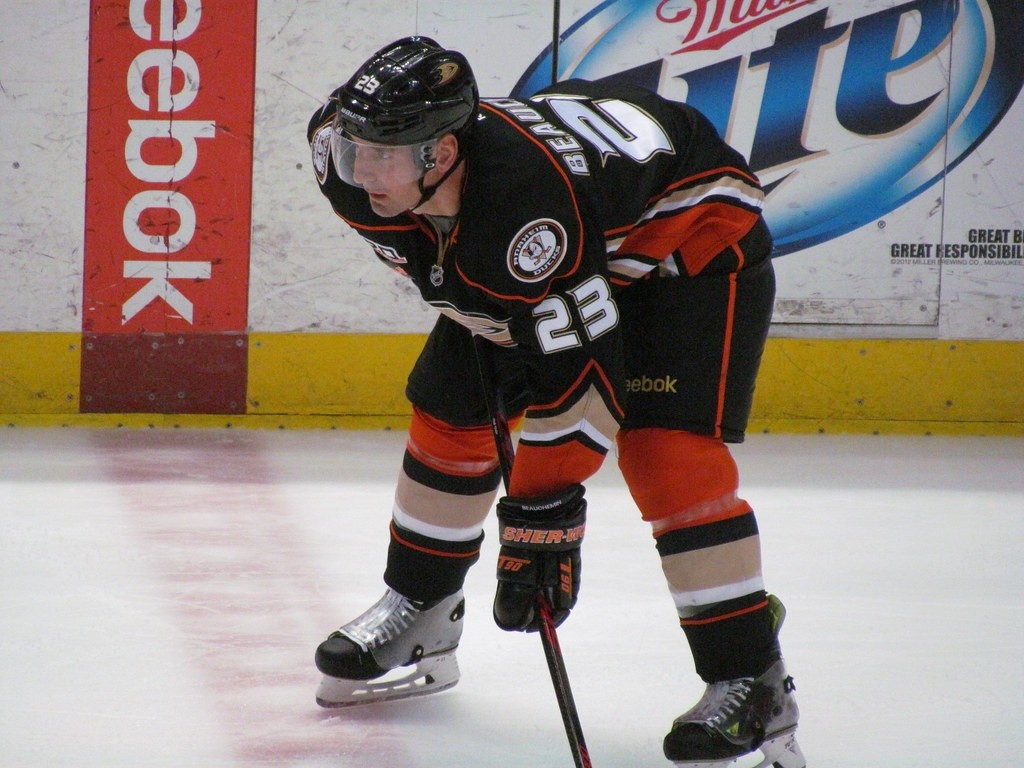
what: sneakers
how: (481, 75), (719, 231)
(663, 594), (806, 768)
(315, 587), (465, 708)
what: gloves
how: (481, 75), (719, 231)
(493, 482), (587, 633)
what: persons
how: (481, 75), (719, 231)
(307, 36), (805, 767)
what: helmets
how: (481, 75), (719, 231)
(337, 35), (479, 169)
